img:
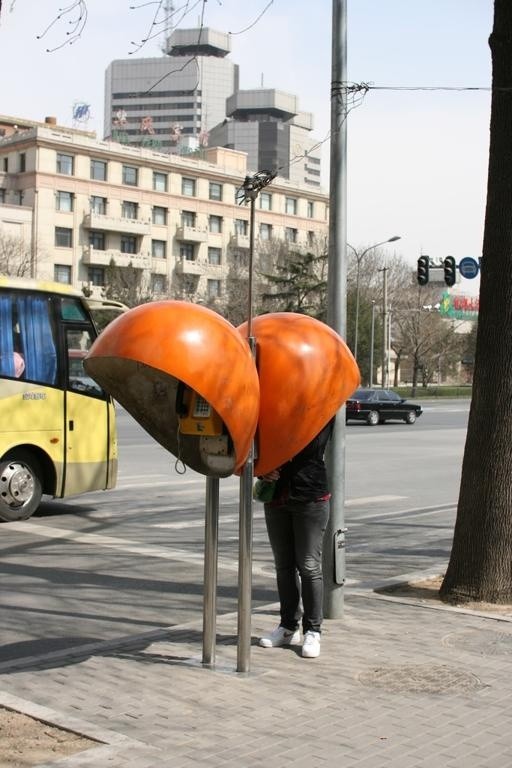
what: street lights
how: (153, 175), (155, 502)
(346, 236), (402, 361)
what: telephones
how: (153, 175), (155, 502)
(174, 381), (223, 436)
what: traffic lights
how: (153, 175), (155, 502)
(417, 255), (429, 286)
(444, 255), (456, 287)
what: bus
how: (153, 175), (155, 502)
(0, 275), (133, 522)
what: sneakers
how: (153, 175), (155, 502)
(300, 630), (322, 660)
(258, 625), (302, 649)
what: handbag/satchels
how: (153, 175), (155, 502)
(253, 477), (281, 504)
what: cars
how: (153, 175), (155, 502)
(346, 388), (424, 425)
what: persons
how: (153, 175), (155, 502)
(260, 413), (335, 659)
